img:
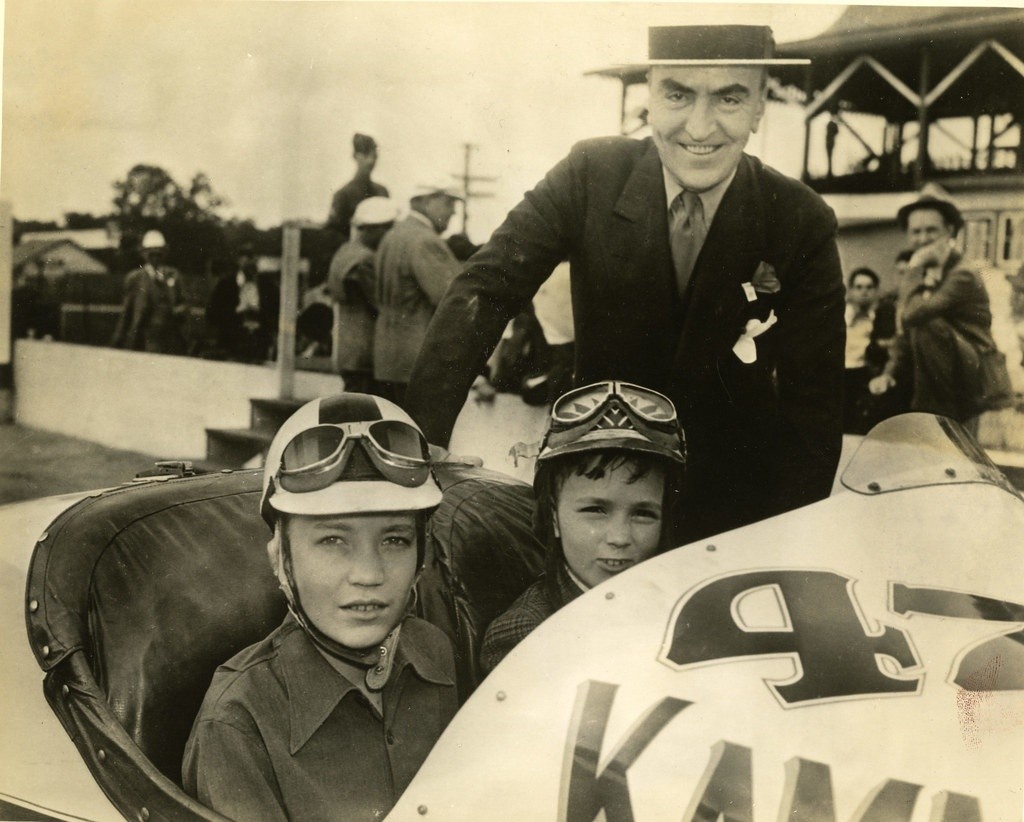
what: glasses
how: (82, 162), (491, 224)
(544, 381), (688, 450)
(276, 420), (434, 494)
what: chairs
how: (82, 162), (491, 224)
(22, 464), (553, 822)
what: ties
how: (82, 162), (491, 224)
(668, 190), (709, 296)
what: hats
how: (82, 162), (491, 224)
(582, 1), (811, 84)
(896, 180), (965, 237)
(352, 197), (398, 228)
(537, 405), (687, 468)
(408, 171), (466, 206)
(257, 391), (442, 522)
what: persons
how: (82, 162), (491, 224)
(183, 392), (465, 822)
(865, 181), (1006, 436)
(845, 251), (915, 436)
(375, 180), (462, 407)
(330, 197), (396, 390)
(209, 268), (279, 363)
(112, 229), (197, 352)
(407, 1), (848, 548)
(329, 133), (388, 233)
(481, 380), (686, 673)
(13, 261), (62, 341)
(472, 312), (536, 402)
